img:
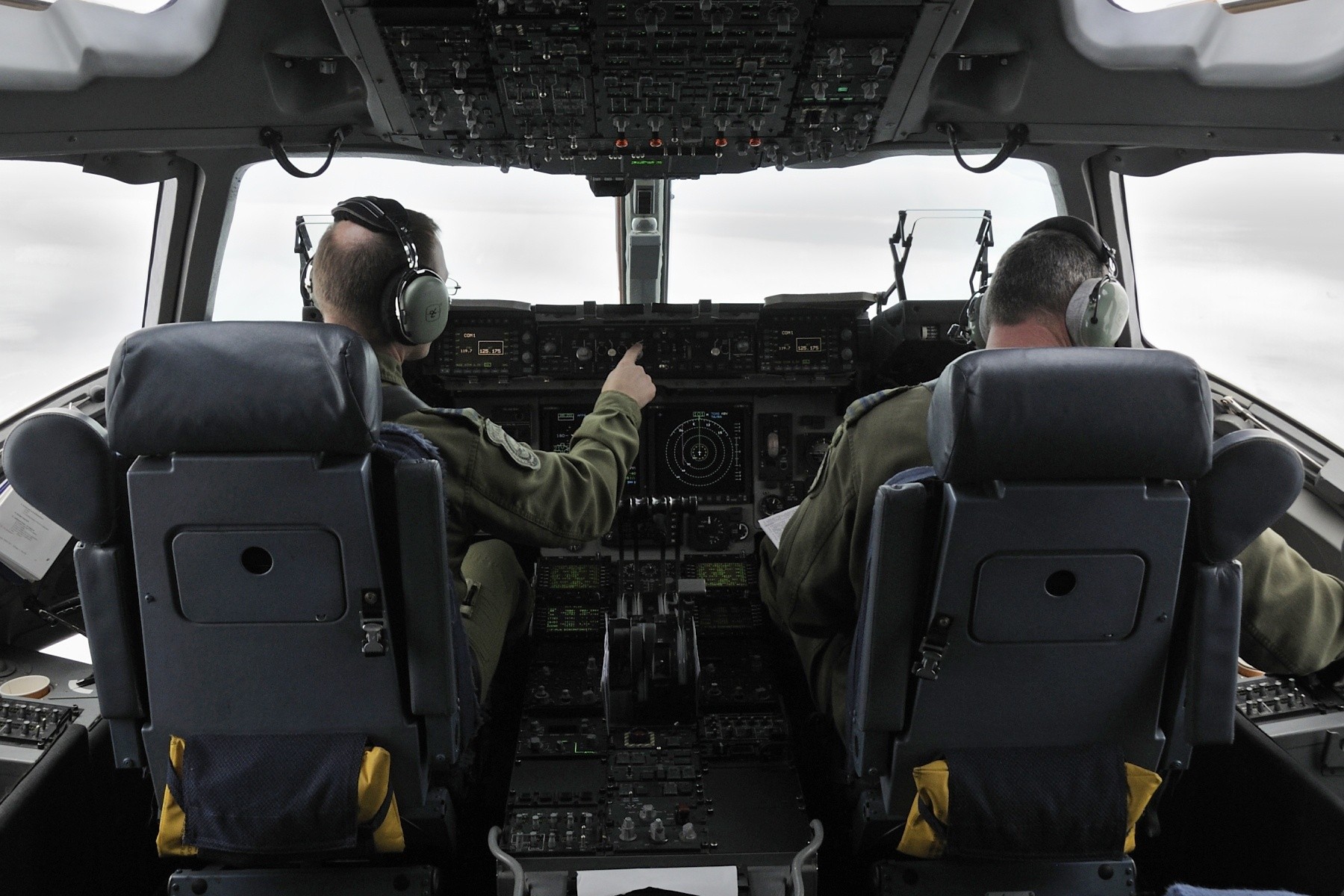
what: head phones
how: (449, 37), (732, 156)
(964, 215), (1130, 348)
(331, 195), (449, 346)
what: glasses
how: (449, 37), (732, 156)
(442, 277), (460, 297)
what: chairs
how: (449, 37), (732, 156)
(3, 321), (479, 862)
(847, 347), (1304, 864)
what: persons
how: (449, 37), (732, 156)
(305, 193), (659, 707)
(758, 227), (1344, 761)
